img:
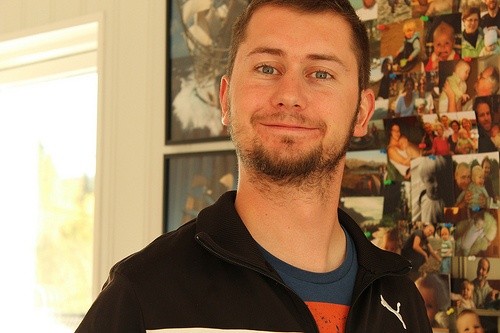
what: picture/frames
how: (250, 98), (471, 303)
(162, 149), (238, 234)
(164, 0), (251, 145)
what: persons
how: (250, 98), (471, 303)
(355, 0), (500, 333)
(75, 0), (433, 333)
